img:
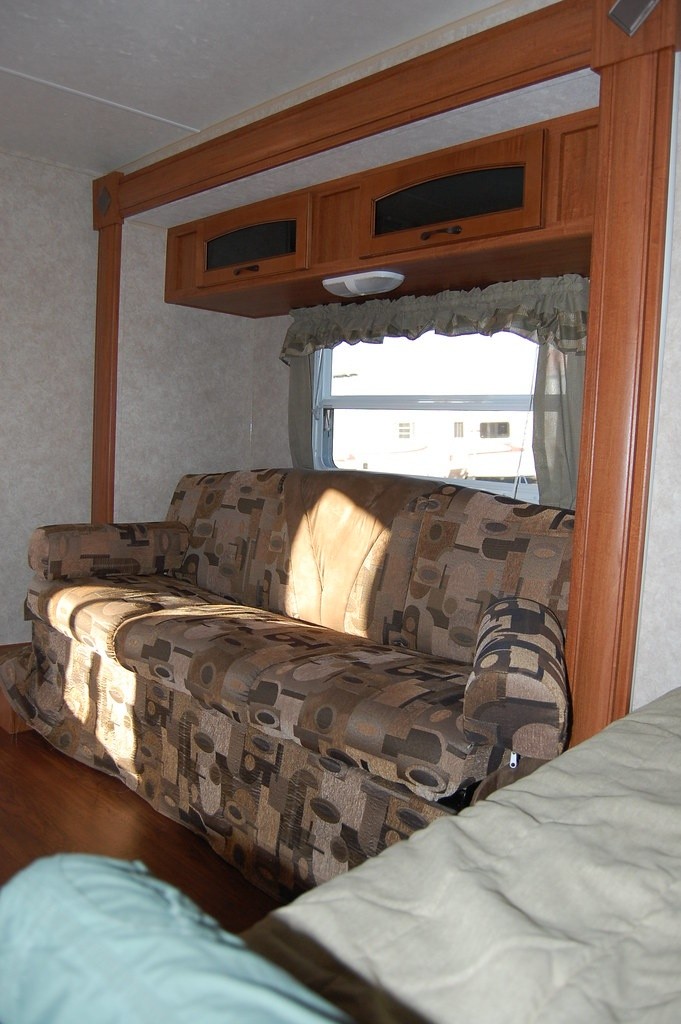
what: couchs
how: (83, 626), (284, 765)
(0, 469), (576, 904)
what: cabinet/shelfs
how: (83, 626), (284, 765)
(159, 107), (599, 318)
